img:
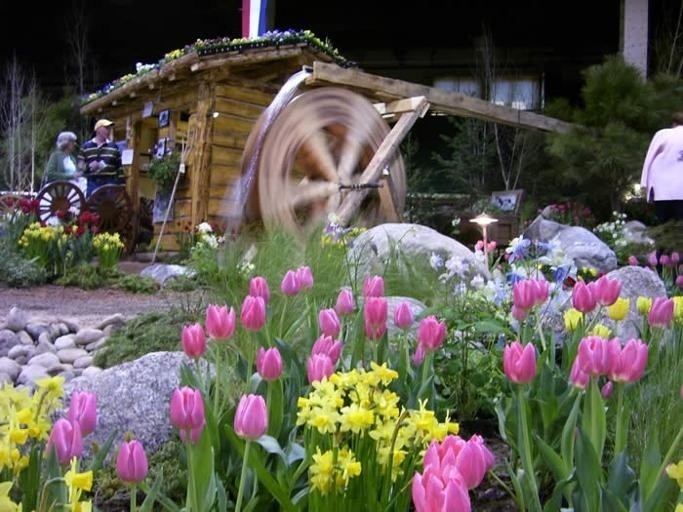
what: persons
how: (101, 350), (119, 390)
(639, 111), (683, 229)
(76, 118), (124, 201)
(43, 130), (86, 188)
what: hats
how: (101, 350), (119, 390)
(93, 118), (116, 131)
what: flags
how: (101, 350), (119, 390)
(242, 0), (266, 38)
